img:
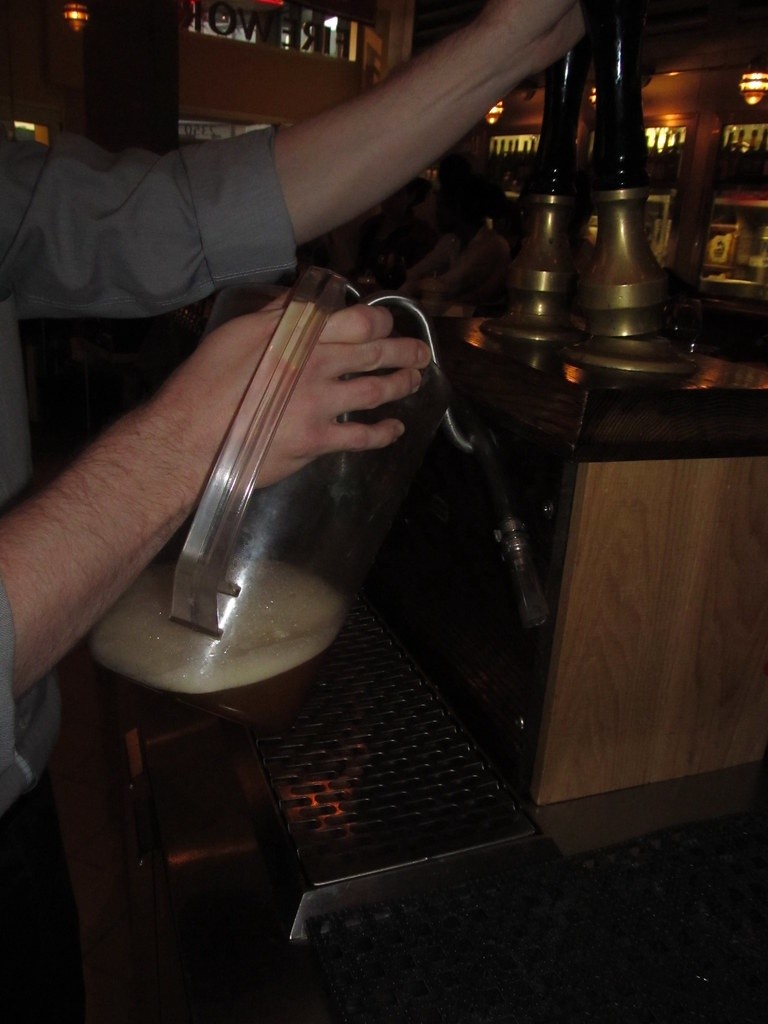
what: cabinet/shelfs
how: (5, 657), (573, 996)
(423, 66), (768, 313)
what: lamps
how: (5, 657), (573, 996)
(738, 53), (768, 106)
(63, 0), (90, 33)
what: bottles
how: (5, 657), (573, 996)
(486, 140), (535, 177)
(716, 127), (768, 183)
(646, 132), (680, 180)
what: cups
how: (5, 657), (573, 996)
(661, 297), (703, 346)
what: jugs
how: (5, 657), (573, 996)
(86, 267), (453, 735)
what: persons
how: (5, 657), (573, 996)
(0, 0), (587, 1023)
